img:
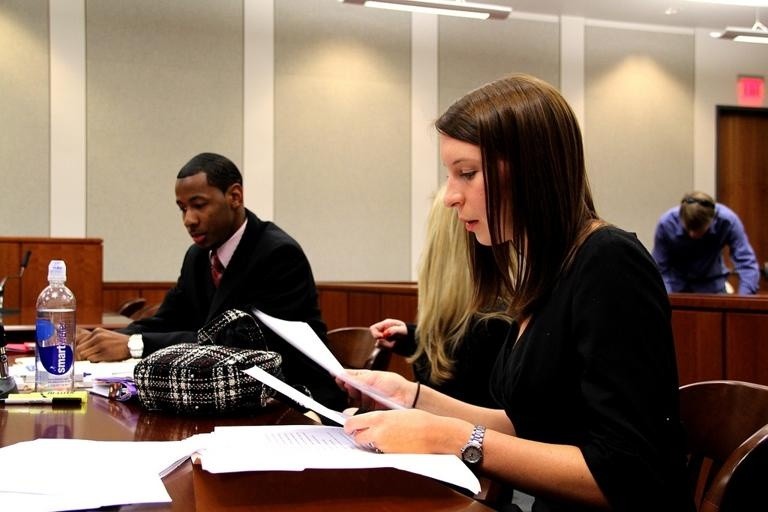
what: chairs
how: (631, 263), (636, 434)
(679, 378), (768, 512)
(115, 297), (147, 315)
(326, 326), (388, 371)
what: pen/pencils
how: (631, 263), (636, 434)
(0, 391), (88, 410)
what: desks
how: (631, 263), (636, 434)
(0, 237), (104, 326)
(664, 291), (767, 374)
(0, 350), (497, 512)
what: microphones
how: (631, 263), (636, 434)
(0, 249), (32, 403)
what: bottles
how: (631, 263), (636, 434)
(31, 259), (78, 396)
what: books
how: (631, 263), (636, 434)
(9, 357), (144, 389)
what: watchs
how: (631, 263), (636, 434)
(460, 424), (486, 464)
(128, 334), (144, 358)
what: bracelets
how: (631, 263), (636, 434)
(412, 381), (420, 408)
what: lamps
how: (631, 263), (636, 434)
(709, 6), (767, 45)
(344, 0), (511, 21)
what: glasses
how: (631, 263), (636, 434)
(682, 198), (714, 207)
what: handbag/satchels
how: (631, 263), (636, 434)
(134, 309), (282, 415)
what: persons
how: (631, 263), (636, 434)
(76, 153), (328, 369)
(370, 182), (513, 410)
(338, 74), (696, 512)
(652, 191), (761, 297)
